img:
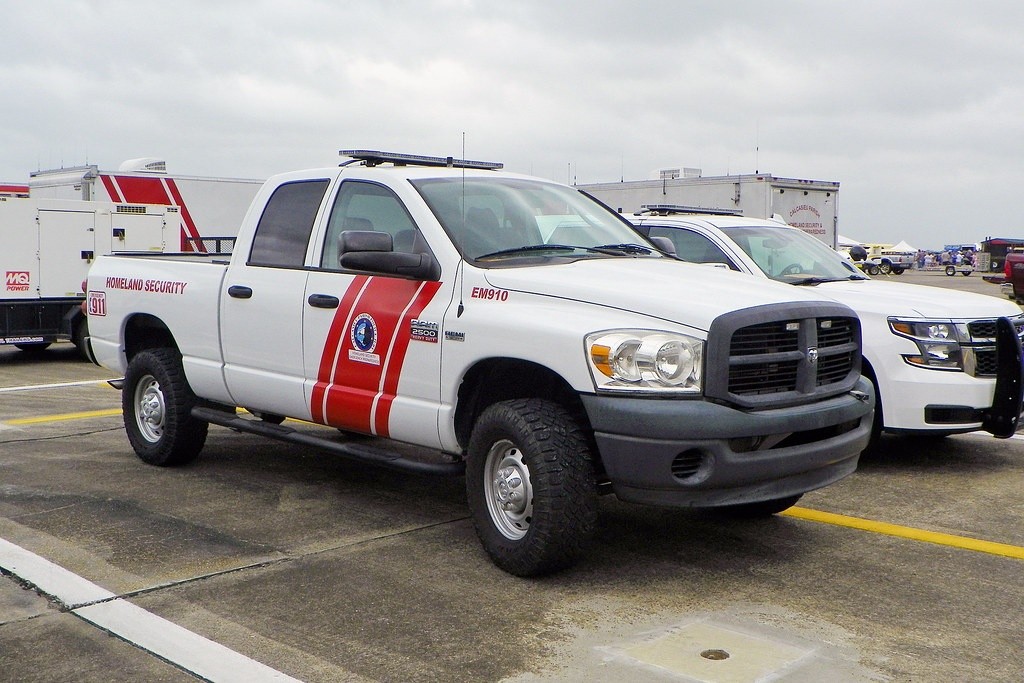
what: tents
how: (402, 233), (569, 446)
(888, 241), (919, 255)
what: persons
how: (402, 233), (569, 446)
(916, 248), (975, 270)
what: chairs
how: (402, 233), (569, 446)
(465, 205), (502, 255)
(395, 228), (417, 252)
(337, 216), (374, 232)
(704, 243), (736, 269)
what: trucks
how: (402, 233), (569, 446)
(0, 164), (416, 367)
(543, 173), (841, 278)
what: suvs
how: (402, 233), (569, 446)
(517, 204), (1024, 464)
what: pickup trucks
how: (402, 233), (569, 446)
(863, 246), (916, 276)
(83, 147), (875, 581)
(1004, 248), (1024, 300)
